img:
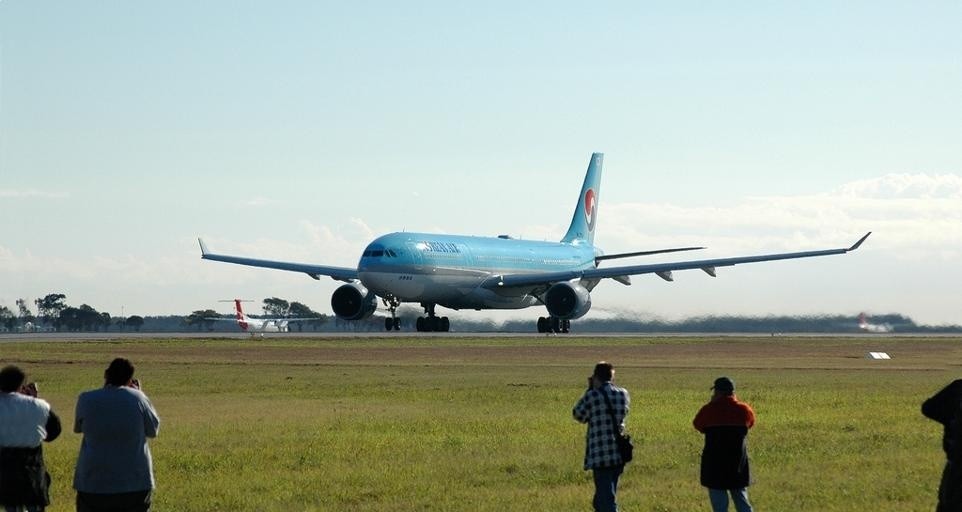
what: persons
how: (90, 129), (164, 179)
(74, 358), (160, 512)
(694, 377), (756, 512)
(922, 379), (962, 512)
(0, 366), (61, 512)
(573, 362), (633, 512)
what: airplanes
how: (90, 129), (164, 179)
(203, 298), (322, 337)
(196, 151), (873, 334)
(834, 309), (912, 335)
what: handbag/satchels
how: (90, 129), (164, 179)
(618, 433), (634, 462)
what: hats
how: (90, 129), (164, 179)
(710, 376), (734, 392)
